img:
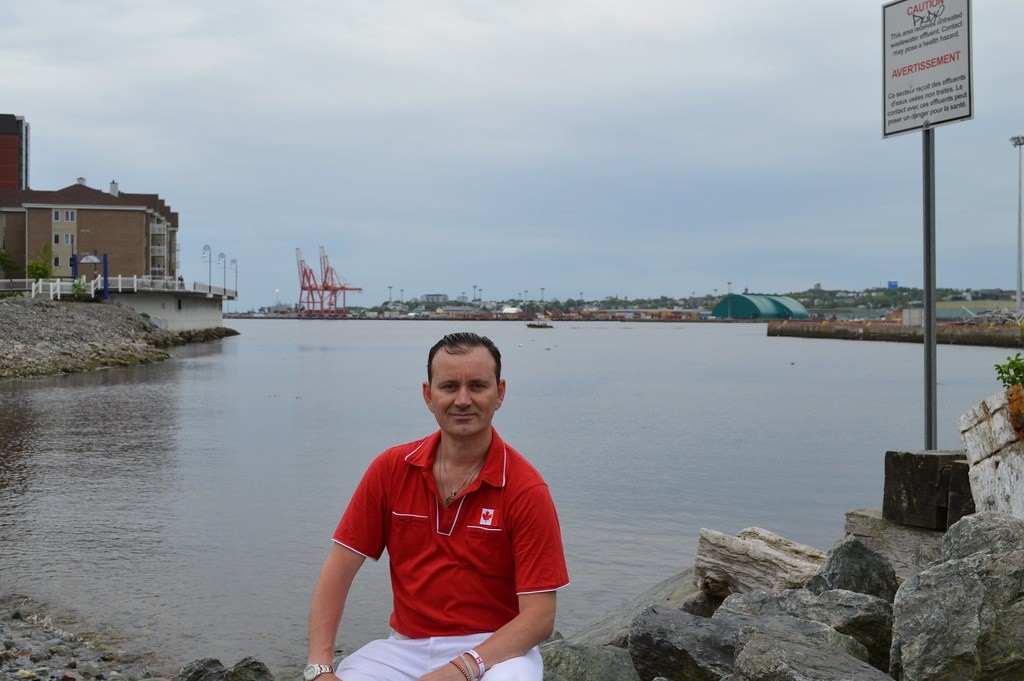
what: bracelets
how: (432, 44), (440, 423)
(450, 650), (485, 681)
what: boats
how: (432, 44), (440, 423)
(526, 321), (553, 328)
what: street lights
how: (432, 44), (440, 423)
(478, 288), (483, 306)
(540, 287), (545, 302)
(201, 245), (212, 293)
(93, 248), (98, 278)
(713, 288), (718, 306)
(274, 288), (280, 318)
(400, 289), (404, 302)
(472, 283), (478, 305)
(518, 292), (522, 302)
(727, 281), (732, 318)
(524, 289), (529, 302)
(388, 286), (393, 302)
(70, 239), (74, 278)
(229, 259), (238, 297)
(217, 253), (227, 296)
(462, 291), (465, 306)
(1010, 136), (1024, 318)
(580, 292), (584, 311)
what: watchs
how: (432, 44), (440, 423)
(303, 664), (335, 681)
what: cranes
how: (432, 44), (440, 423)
(295, 246), (361, 316)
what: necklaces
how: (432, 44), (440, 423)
(439, 447), (482, 508)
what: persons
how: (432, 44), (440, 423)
(307, 331), (571, 681)
(830, 314), (837, 322)
(178, 275), (183, 281)
